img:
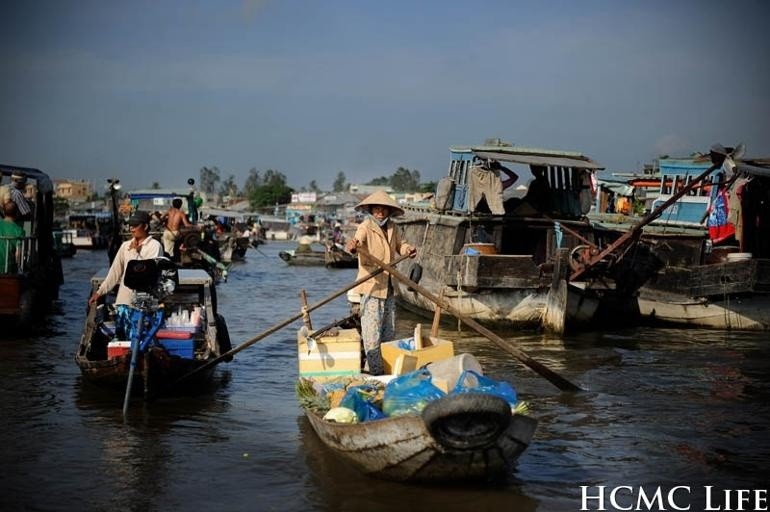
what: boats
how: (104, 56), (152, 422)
(0, 160), (66, 335)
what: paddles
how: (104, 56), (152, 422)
(355, 246), (583, 392)
(569, 145), (746, 280)
(178, 251), (411, 378)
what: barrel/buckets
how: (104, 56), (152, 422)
(463, 243), (497, 256)
(420, 352), (483, 388)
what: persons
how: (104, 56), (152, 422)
(523, 163), (554, 216)
(88, 209), (165, 340)
(0, 169), (38, 280)
(148, 176), (261, 259)
(344, 189), (418, 376)
(606, 191), (634, 215)
(297, 210), (347, 261)
(474, 159), (522, 215)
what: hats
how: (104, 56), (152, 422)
(122, 214), (150, 227)
(353, 188), (405, 217)
